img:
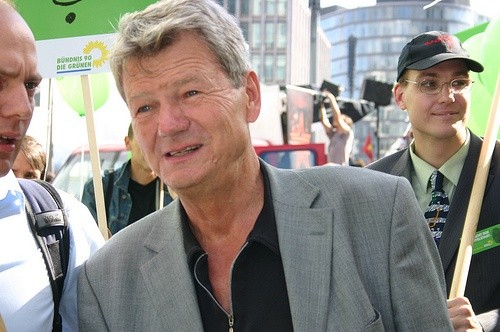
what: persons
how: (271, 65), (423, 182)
(0, 0), (107, 332)
(11, 135), (47, 180)
(77, 2), (454, 332)
(361, 31), (500, 332)
(318, 90), (354, 167)
(81, 122), (177, 241)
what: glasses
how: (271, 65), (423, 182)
(399, 77), (475, 96)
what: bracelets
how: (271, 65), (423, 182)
(478, 320), (485, 332)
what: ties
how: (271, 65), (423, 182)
(423, 171), (450, 248)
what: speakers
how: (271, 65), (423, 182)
(361, 79), (393, 106)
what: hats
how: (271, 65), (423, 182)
(396, 30), (485, 83)
